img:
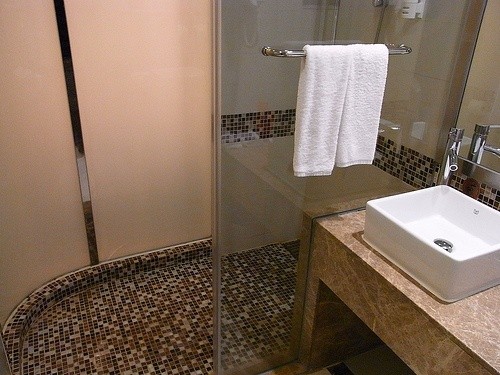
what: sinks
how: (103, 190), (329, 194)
(364, 184), (500, 303)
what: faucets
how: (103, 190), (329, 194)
(466, 123), (500, 164)
(435, 126), (465, 185)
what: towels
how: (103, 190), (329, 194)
(291, 43), (389, 177)
